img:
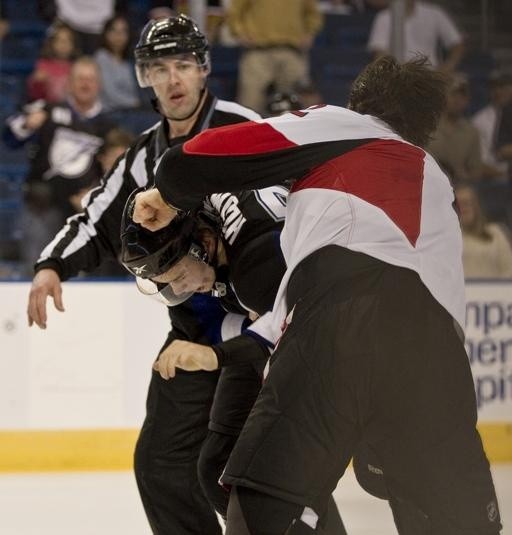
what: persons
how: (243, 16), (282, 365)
(150, 49), (505, 532)
(228, 0), (510, 281)
(4, 0), (239, 281)
(114, 172), (388, 499)
(19, 12), (349, 532)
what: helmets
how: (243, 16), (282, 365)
(134, 12), (211, 88)
(120, 186), (221, 307)
